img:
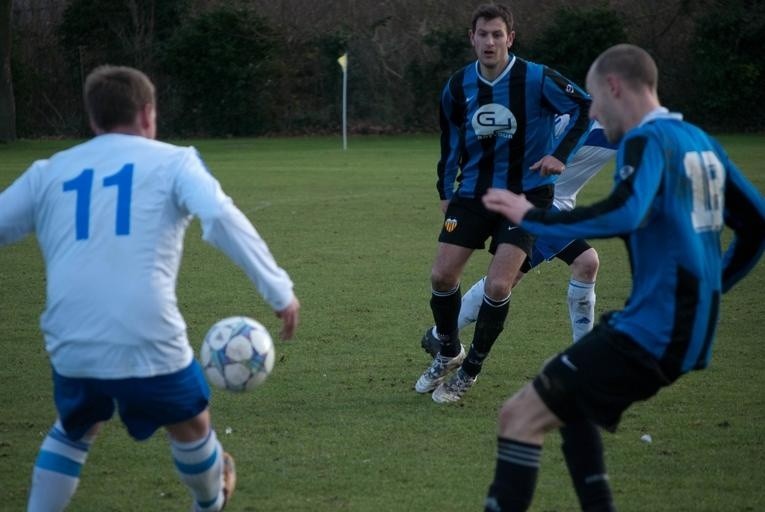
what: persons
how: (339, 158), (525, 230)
(414, 5), (592, 402)
(1, 66), (300, 511)
(422, 60), (620, 358)
(483, 43), (764, 512)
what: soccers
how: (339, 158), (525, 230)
(202, 316), (274, 392)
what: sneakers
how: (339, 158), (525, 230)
(421, 326), (441, 359)
(219, 448), (238, 511)
(431, 367), (481, 407)
(411, 342), (469, 393)
